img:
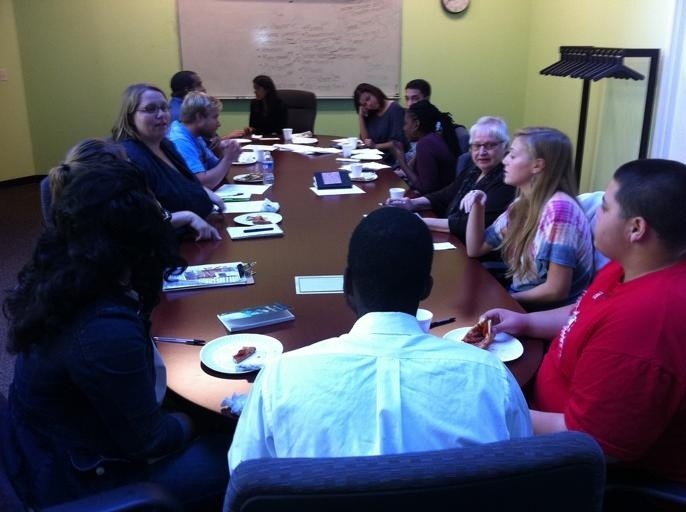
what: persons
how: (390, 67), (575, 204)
(224, 202), (538, 472)
(51, 134), (167, 304)
(0, 164), (208, 508)
(75, 67), (519, 254)
(476, 155), (686, 490)
(457, 127), (596, 313)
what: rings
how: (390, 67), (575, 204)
(470, 190), (475, 193)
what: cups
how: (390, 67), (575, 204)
(342, 137), (358, 162)
(351, 163), (362, 177)
(282, 128), (293, 140)
(417, 308), (434, 334)
(388, 188), (405, 200)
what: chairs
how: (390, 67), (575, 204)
(615, 473), (685, 512)
(454, 124), (471, 154)
(480, 189), (612, 281)
(38, 173), (56, 230)
(273, 89), (318, 134)
(212, 429), (608, 512)
(454, 150), (472, 180)
(0, 392), (179, 511)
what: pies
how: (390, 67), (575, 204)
(233, 347), (256, 365)
(460, 316), (496, 350)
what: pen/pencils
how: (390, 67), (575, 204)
(152, 337), (206, 344)
(244, 228), (273, 232)
(430, 318), (455, 328)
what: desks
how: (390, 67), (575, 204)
(149, 133), (547, 419)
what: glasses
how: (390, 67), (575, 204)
(135, 103), (168, 113)
(468, 140), (506, 152)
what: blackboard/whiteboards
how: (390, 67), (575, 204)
(177, 0), (401, 100)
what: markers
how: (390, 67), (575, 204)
(237, 96), (248, 99)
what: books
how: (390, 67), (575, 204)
(213, 302), (298, 332)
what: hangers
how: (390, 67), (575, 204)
(537, 44), (646, 84)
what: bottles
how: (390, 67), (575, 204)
(263, 152), (276, 187)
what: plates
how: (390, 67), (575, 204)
(202, 335), (282, 374)
(350, 154), (383, 161)
(234, 173), (274, 182)
(294, 138), (318, 145)
(233, 211), (283, 225)
(226, 137), (264, 166)
(349, 172), (378, 183)
(443, 324), (523, 363)
